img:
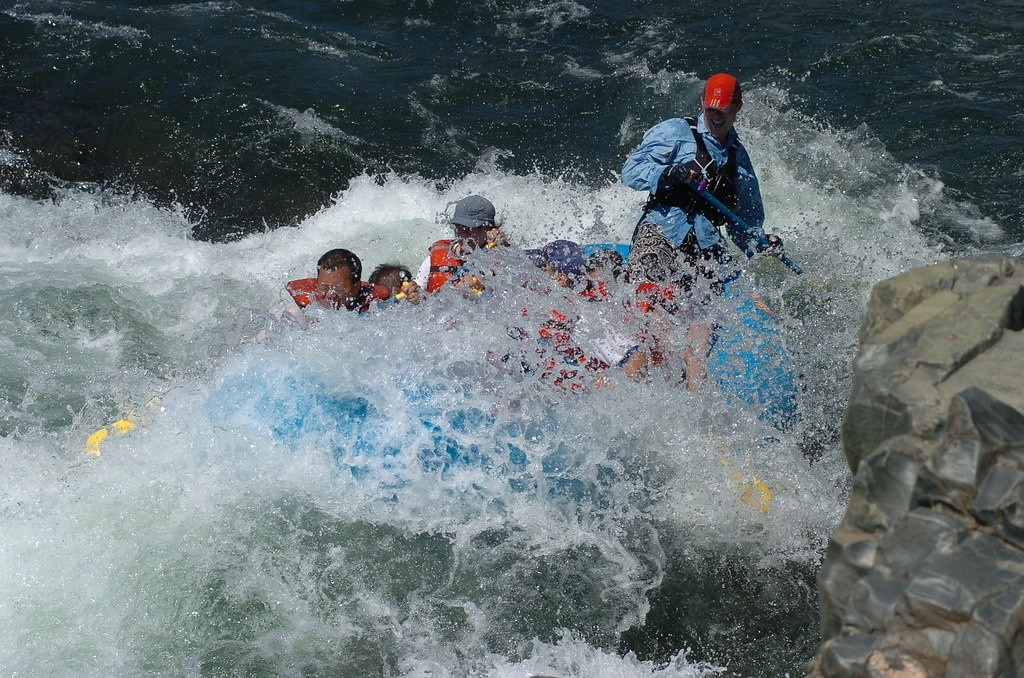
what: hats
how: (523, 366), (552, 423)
(449, 195), (496, 229)
(702, 73), (743, 114)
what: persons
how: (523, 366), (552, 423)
(259, 196), (630, 395)
(594, 72), (784, 397)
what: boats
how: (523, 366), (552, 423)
(209, 241), (796, 525)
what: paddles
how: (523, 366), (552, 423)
(667, 157), (804, 275)
